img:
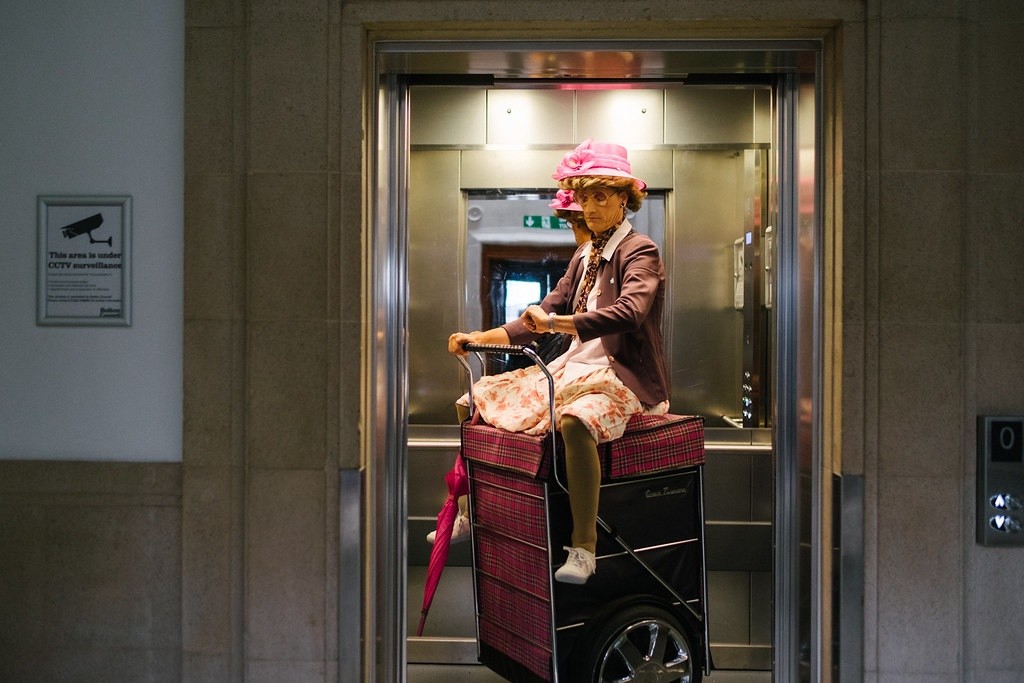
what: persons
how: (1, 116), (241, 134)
(510, 191), (587, 374)
(421, 139), (671, 582)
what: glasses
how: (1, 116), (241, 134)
(566, 219), (575, 229)
(572, 189), (618, 206)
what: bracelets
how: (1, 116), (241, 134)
(548, 313), (557, 334)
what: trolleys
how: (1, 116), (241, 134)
(453, 342), (714, 683)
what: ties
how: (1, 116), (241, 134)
(572, 220), (624, 339)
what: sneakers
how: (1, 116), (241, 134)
(426, 505), (470, 545)
(555, 546), (596, 584)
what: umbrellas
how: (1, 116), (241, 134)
(417, 407), (480, 637)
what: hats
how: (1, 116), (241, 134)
(548, 188), (583, 212)
(552, 139), (646, 192)
